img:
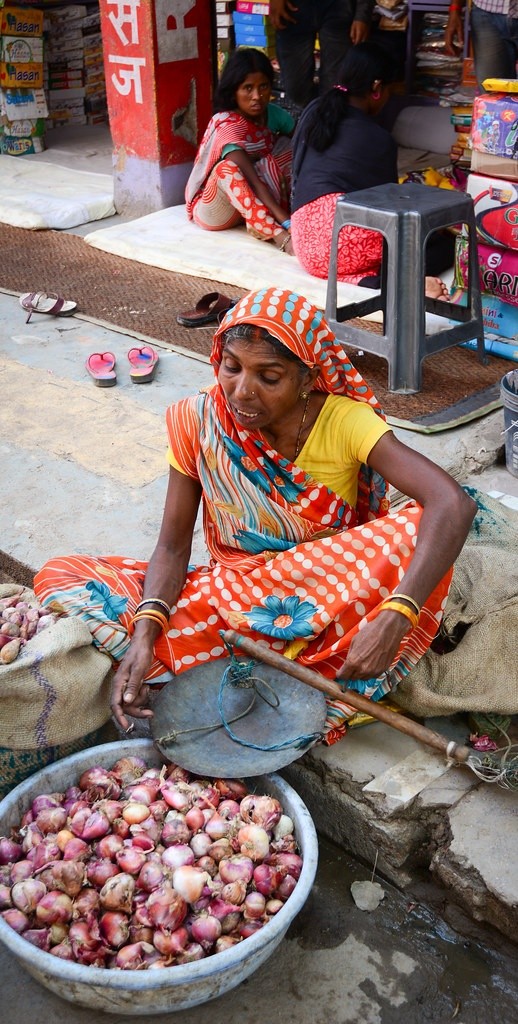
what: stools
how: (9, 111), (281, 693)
(325, 183), (488, 391)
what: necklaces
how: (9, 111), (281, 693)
(294, 395), (311, 462)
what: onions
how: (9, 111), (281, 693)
(0, 756), (301, 970)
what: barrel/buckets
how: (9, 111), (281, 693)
(500, 368), (518, 478)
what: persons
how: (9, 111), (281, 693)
(290, 42), (452, 302)
(444, 0), (518, 95)
(184, 48), (294, 255)
(30, 285), (478, 748)
(268, 0), (375, 110)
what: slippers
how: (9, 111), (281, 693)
(127, 346), (159, 383)
(86, 351), (117, 387)
(213, 308), (231, 325)
(176, 292), (239, 328)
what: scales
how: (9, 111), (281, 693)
(151, 630), (473, 779)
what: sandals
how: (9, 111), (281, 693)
(19, 291), (78, 324)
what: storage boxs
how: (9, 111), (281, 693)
(216, 0), (280, 64)
(0, 7), (108, 155)
(450, 96), (518, 359)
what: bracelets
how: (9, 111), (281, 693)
(280, 218), (291, 230)
(448, 4), (461, 12)
(127, 609), (168, 630)
(133, 597), (171, 614)
(379, 592), (422, 628)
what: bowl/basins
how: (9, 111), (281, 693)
(0, 736), (318, 1016)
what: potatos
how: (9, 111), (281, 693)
(0, 594), (58, 665)
